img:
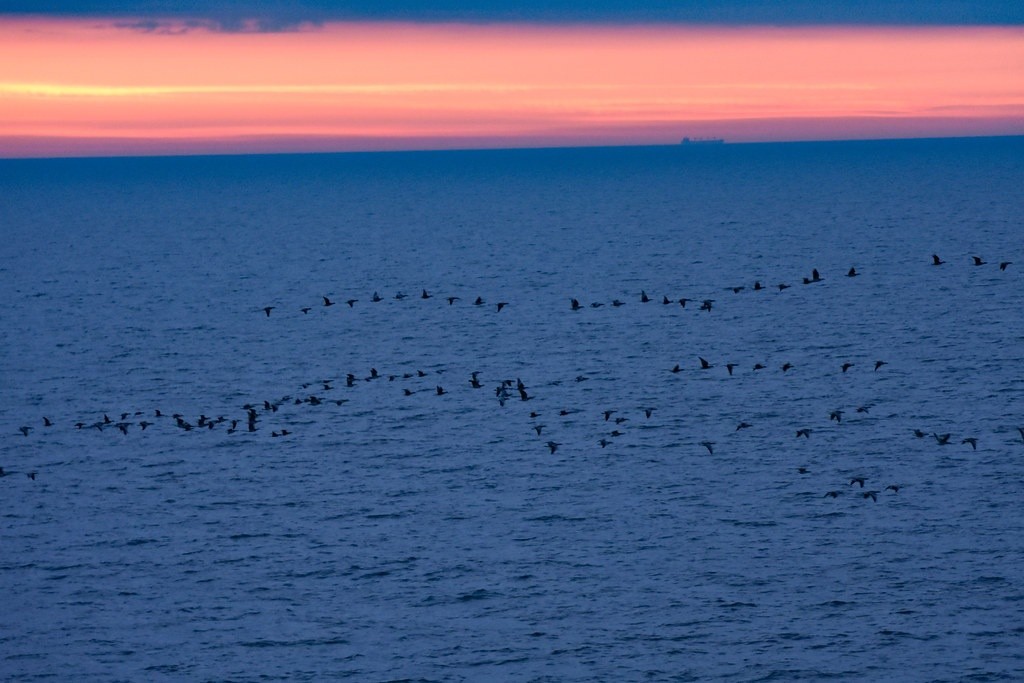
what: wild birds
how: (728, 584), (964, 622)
(570, 288), (717, 313)
(0, 367), (449, 480)
(971, 256), (987, 266)
(467, 371), (657, 455)
(669, 357), (978, 502)
(735, 266), (861, 294)
(931, 254), (946, 265)
(263, 288), (509, 317)
(999, 262), (1013, 271)
